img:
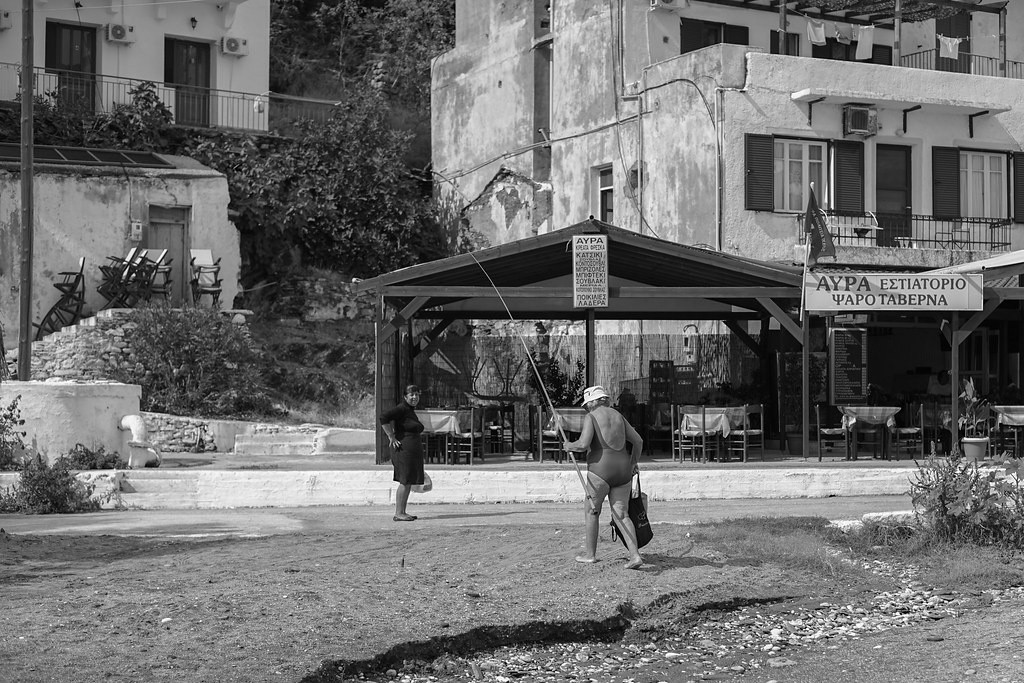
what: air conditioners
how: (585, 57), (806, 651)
(844, 106), (878, 135)
(0, 10), (12, 28)
(106, 22), (135, 44)
(221, 36), (249, 54)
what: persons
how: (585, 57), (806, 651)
(561, 384), (645, 568)
(380, 384), (426, 521)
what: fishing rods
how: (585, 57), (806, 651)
(464, 250), (599, 516)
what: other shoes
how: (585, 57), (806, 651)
(392, 517), (414, 521)
(412, 516), (417, 519)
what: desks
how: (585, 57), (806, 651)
(545, 407), (591, 461)
(990, 406), (1024, 459)
(414, 409), (480, 464)
(681, 408), (750, 461)
(837, 404), (901, 461)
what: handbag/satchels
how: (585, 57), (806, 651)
(632, 474), (647, 515)
(411, 472), (432, 493)
(610, 471), (654, 551)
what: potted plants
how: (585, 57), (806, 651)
(958, 377), (991, 460)
(779, 354), (822, 455)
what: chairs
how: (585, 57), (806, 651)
(189, 248), (224, 309)
(529, 404), (569, 463)
(886, 402), (924, 458)
(646, 402), (764, 462)
(817, 405), (849, 461)
(414, 402), (514, 464)
(97, 246), (174, 310)
(53, 257), (87, 326)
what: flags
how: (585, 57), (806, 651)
(803, 183), (837, 266)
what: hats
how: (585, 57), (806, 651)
(581, 385), (609, 407)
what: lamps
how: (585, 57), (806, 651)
(191, 17), (199, 29)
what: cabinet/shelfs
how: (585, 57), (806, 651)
(650, 0), (685, 10)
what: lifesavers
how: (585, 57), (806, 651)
(410, 471), (432, 493)
